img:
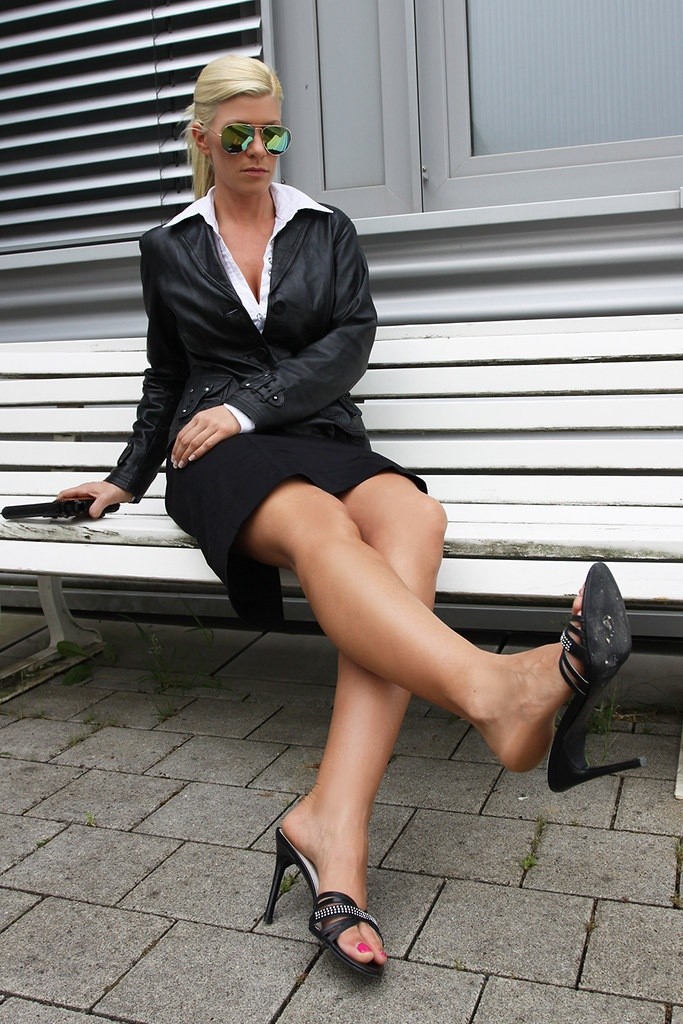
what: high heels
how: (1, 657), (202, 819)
(263, 828), (385, 978)
(546, 561), (646, 793)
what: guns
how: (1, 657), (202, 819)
(1, 498), (121, 520)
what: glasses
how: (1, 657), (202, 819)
(194, 120), (293, 158)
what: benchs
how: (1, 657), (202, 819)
(1, 316), (682, 801)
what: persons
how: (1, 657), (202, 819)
(55, 57), (645, 983)
